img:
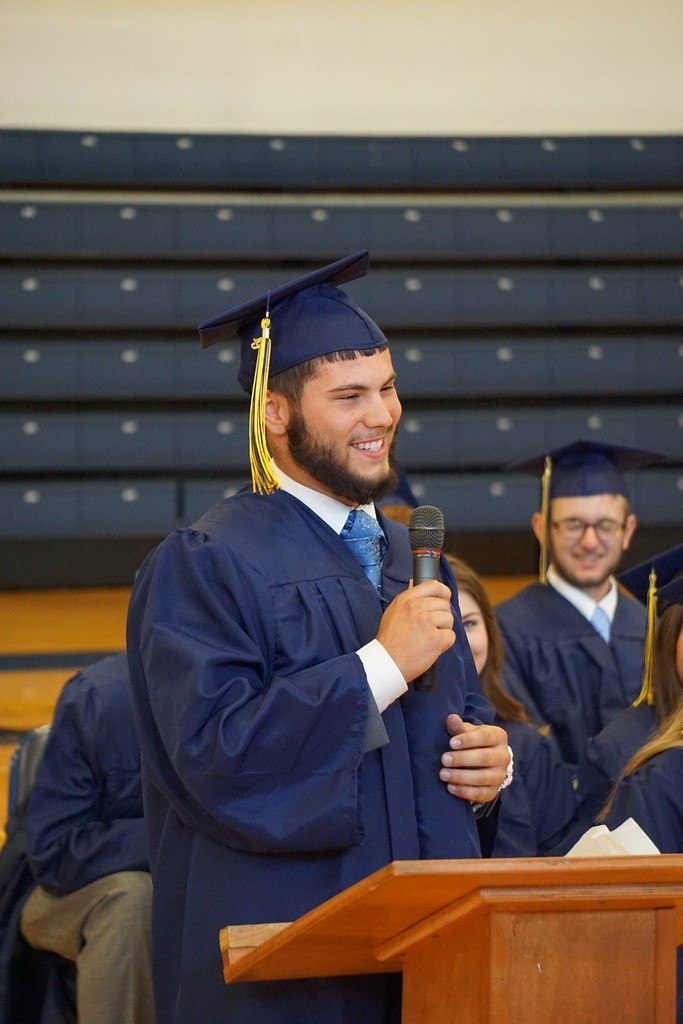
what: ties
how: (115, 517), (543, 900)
(343, 508), (389, 600)
(592, 605), (609, 644)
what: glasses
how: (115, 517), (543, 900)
(550, 518), (624, 539)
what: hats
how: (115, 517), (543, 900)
(507, 436), (668, 585)
(198, 247), (389, 496)
(615, 546), (682, 708)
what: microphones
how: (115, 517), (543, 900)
(407, 507), (445, 693)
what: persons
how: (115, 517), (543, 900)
(1, 640), (163, 1023)
(426, 553), (588, 861)
(123, 303), (517, 1024)
(588, 602), (683, 859)
(476, 466), (669, 858)
